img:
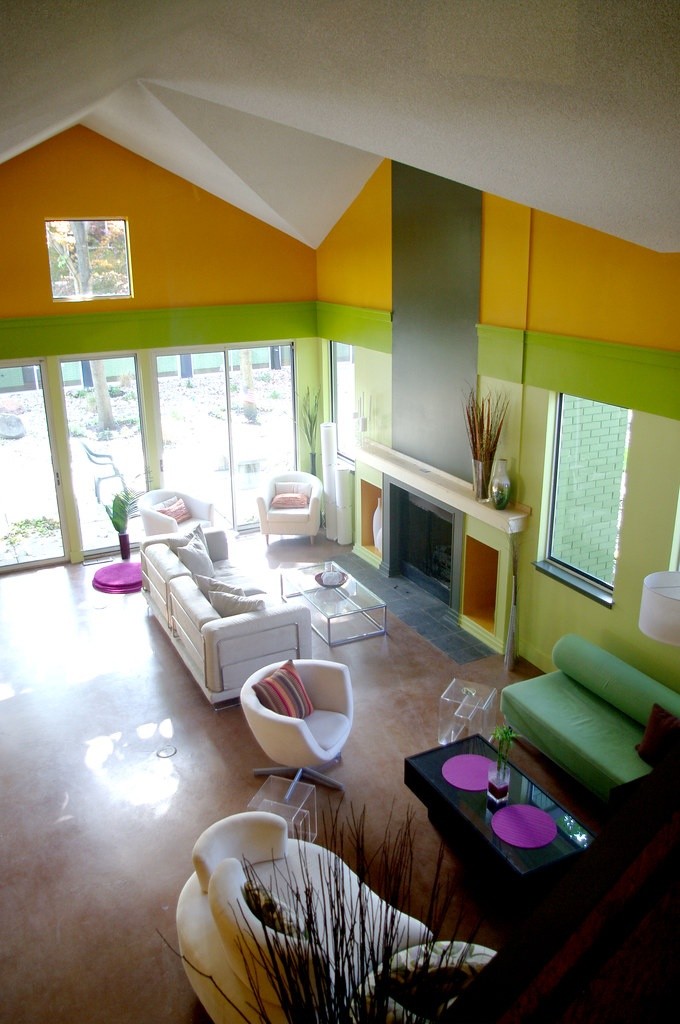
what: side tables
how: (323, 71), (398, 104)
(435, 678), (499, 746)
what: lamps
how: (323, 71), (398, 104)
(637, 570), (680, 646)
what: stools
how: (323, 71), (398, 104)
(245, 776), (320, 843)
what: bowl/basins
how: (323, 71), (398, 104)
(314, 570), (348, 589)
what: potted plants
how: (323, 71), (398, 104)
(457, 380), (513, 504)
(486, 721), (524, 803)
(101, 486), (151, 559)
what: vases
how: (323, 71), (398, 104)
(310, 452), (323, 528)
(488, 457), (512, 511)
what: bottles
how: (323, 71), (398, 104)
(490, 459), (511, 510)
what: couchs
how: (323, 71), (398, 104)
(136, 525), (315, 712)
(498, 644), (680, 793)
(166, 808), (499, 1024)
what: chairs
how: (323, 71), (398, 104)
(71, 436), (132, 505)
(137, 488), (217, 536)
(255, 470), (324, 546)
(245, 652), (358, 800)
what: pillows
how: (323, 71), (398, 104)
(252, 657), (315, 716)
(154, 495), (179, 510)
(194, 572), (246, 601)
(177, 534), (217, 578)
(168, 522), (211, 559)
(158, 498), (191, 523)
(275, 482), (311, 503)
(242, 878), (308, 940)
(271, 491), (308, 508)
(635, 703), (680, 770)
(206, 590), (266, 617)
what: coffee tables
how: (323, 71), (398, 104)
(279, 558), (391, 649)
(402, 732), (601, 882)
